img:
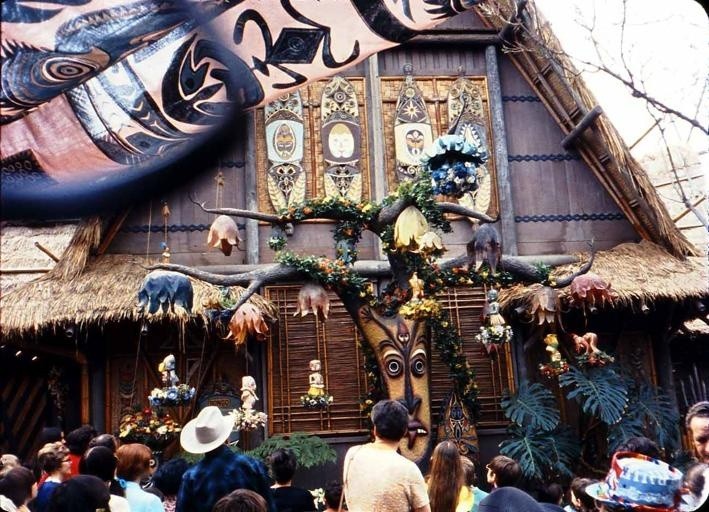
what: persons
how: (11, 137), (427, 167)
(0, 398), (709, 511)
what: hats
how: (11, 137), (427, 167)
(584, 451), (709, 512)
(180, 405), (235, 455)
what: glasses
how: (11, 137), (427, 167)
(486, 464), (493, 473)
(60, 457), (71, 464)
(594, 499), (601, 511)
